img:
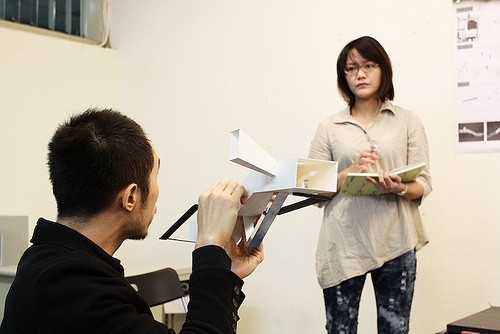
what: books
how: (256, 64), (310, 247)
(340, 163), (426, 195)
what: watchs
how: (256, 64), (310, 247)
(397, 185), (408, 196)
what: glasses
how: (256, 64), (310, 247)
(344, 64), (379, 75)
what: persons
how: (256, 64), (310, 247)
(307, 36), (433, 334)
(0, 106), (264, 334)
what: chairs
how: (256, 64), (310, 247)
(125, 268), (188, 316)
